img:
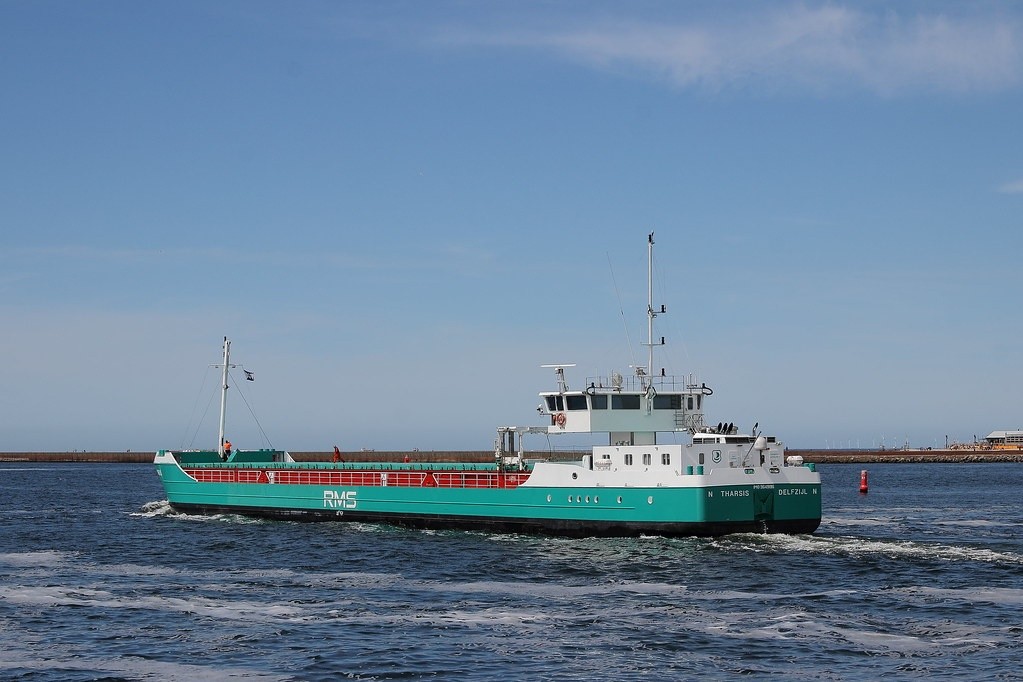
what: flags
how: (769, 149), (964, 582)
(244, 369), (256, 382)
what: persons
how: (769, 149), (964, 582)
(223, 440), (231, 461)
(333, 445), (345, 464)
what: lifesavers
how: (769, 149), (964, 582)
(556, 413), (565, 426)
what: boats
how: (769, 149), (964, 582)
(151, 232), (824, 542)
